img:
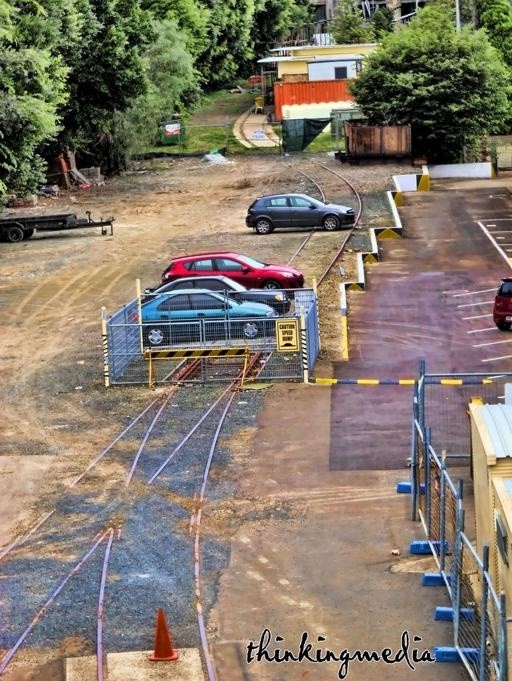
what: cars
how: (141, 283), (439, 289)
(134, 292), (278, 346)
(247, 195), (355, 232)
(162, 251), (303, 293)
(493, 277), (512, 332)
(143, 277), (290, 313)
